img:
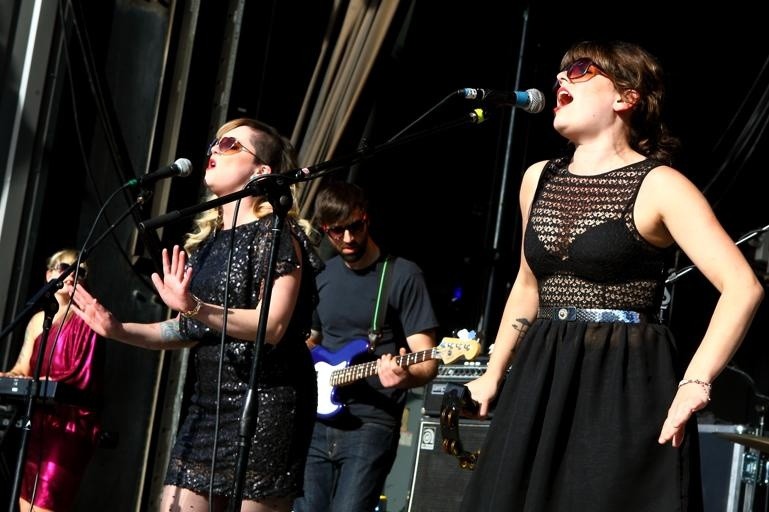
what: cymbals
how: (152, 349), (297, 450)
(717, 432), (769, 452)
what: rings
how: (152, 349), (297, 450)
(81, 307), (86, 313)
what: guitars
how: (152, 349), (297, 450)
(310, 329), (481, 419)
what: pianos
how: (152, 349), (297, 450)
(0, 376), (99, 412)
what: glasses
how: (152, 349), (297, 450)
(549, 57), (615, 87)
(54, 262), (88, 280)
(325, 213), (369, 241)
(206, 137), (262, 158)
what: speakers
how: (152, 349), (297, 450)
(408, 418), (492, 512)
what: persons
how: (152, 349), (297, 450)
(455, 28), (767, 511)
(0, 247), (120, 512)
(68, 117), (330, 511)
(286, 181), (443, 512)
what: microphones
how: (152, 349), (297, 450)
(463, 88), (546, 113)
(125, 157), (192, 187)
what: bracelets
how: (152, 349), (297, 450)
(677, 377), (713, 401)
(180, 291), (202, 318)
